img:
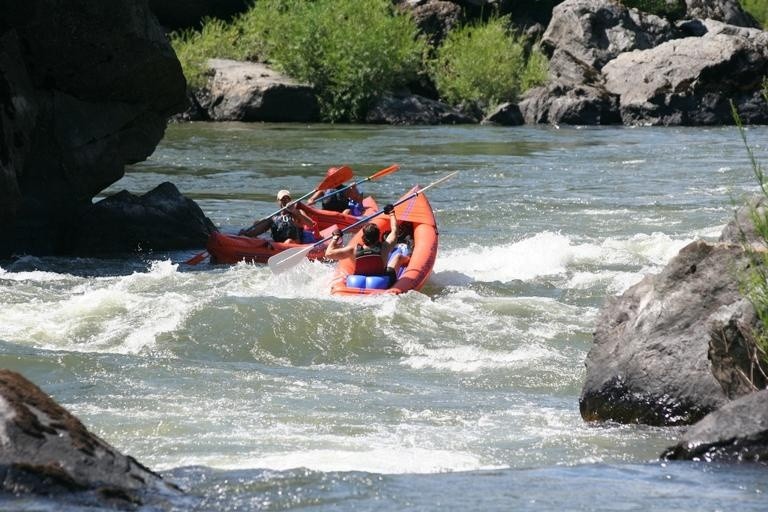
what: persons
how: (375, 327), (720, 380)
(306, 167), (363, 215)
(324, 204), (411, 289)
(238, 189), (314, 244)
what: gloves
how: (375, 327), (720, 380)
(384, 204), (394, 214)
(332, 230), (343, 240)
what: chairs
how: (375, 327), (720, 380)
(354, 255), (385, 276)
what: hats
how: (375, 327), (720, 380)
(363, 224), (379, 242)
(277, 190), (291, 200)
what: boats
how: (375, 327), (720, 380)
(330, 186), (440, 296)
(208, 194), (378, 266)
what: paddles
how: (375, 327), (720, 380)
(186, 166), (353, 264)
(307, 163), (400, 206)
(268, 171), (459, 276)
